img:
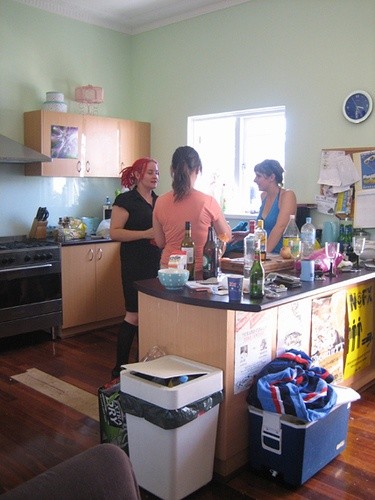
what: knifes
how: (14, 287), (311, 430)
(36, 207), (49, 221)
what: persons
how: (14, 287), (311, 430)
(109, 158), (164, 380)
(231, 159), (297, 254)
(152, 145), (230, 281)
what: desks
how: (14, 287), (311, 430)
(133, 261), (375, 478)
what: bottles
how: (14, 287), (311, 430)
(181, 221), (195, 281)
(282, 214), (301, 247)
(202, 226), (218, 280)
(168, 375), (188, 388)
(232, 219), (267, 299)
(104, 196), (112, 205)
(339, 212), (353, 242)
(55, 216), (86, 242)
(301, 218), (317, 256)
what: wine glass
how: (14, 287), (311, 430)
(289, 240), (301, 273)
(352, 237), (366, 271)
(325, 241), (340, 278)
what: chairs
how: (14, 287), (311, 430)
(0, 443), (142, 500)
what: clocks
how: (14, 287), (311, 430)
(341, 89), (373, 124)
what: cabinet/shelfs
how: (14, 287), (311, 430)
(42, 236), (127, 340)
(119, 118), (151, 178)
(23, 110), (121, 178)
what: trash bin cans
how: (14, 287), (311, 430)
(120, 354), (223, 500)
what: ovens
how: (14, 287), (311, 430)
(0, 260), (62, 336)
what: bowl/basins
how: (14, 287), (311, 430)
(157, 268), (189, 290)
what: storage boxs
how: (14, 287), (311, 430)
(75, 217), (101, 235)
(247, 398), (352, 492)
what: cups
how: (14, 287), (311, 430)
(228, 274), (245, 301)
(301, 259), (315, 281)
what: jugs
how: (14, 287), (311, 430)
(321, 220), (339, 246)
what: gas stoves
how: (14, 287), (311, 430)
(0, 235), (62, 268)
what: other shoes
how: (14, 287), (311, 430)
(112, 369), (120, 381)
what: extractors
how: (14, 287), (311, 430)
(0, 134), (52, 163)
